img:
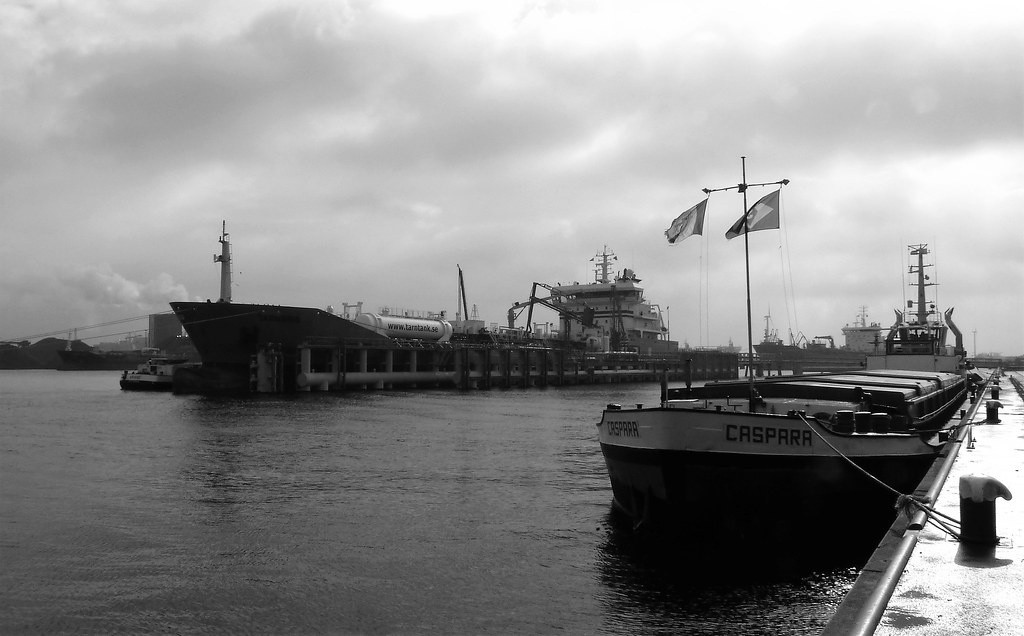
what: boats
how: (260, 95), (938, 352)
(168, 214), (679, 398)
(120, 346), (202, 391)
(596, 150), (995, 513)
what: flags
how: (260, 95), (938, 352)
(664, 198), (708, 246)
(725, 190), (780, 240)
